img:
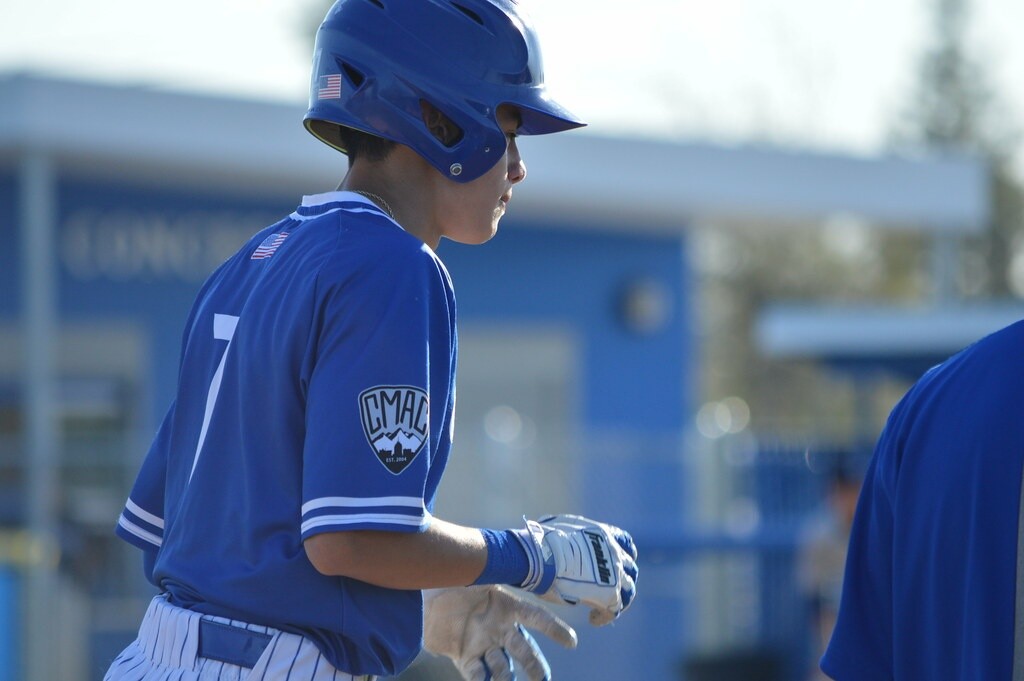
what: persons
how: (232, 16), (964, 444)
(820, 319), (1023, 681)
(101, 0), (638, 681)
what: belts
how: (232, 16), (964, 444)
(196, 618), (274, 669)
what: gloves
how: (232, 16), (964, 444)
(523, 513), (642, 626)
(422, 585), (577, 681)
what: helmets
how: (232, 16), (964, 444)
(304, 0), (589, 185)
